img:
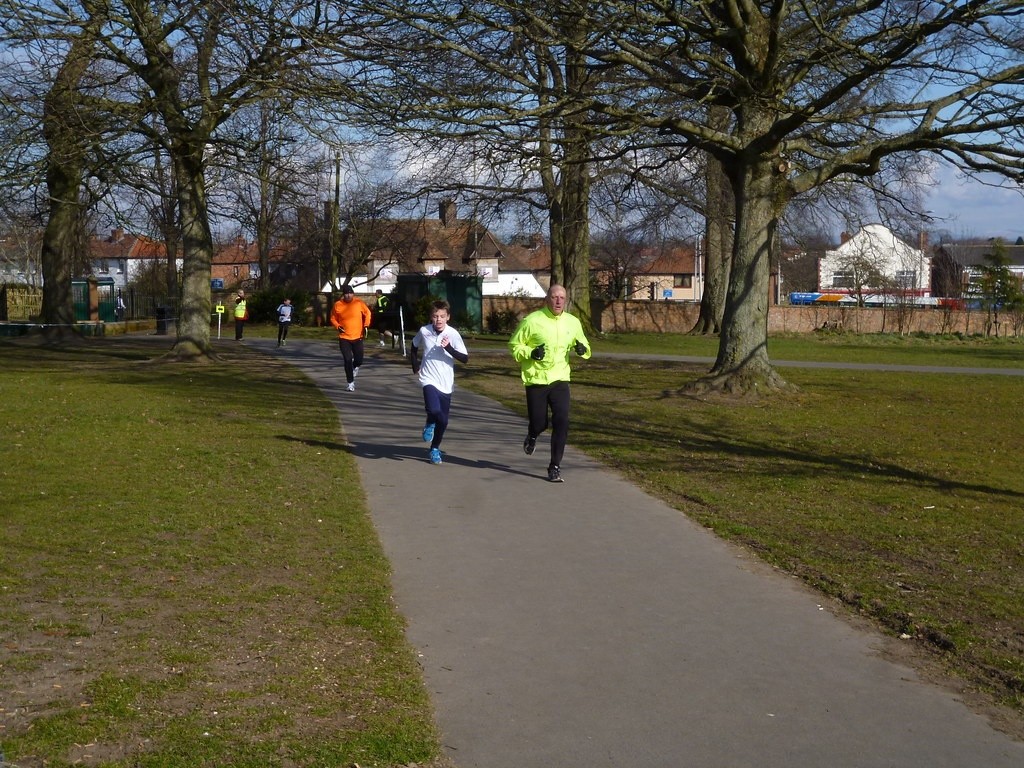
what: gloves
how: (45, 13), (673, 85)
(531, 343), (546, 361)
(573, 339), (587, 355)
(337, 326), (345, 334)
(363, 326), (369, 339)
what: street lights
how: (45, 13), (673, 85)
(919, 210), (933, 296)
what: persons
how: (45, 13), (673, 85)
(375, 290), (399, 347)
(330, 285), (371, 392)
(114, 294), (126, 320)
(234, 289), (246, 341)
(411, 300), (468, 465)
(277, 299), (294, 348)
(508, 284), (591, 483)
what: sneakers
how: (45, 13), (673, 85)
(547, 464), (564, 482)
(346, 382), (355, 392)
(523, 433), (537, 456)
(428, 447), (442, 465)
(422, 422), (435, 442)
(352, 366), (360, 377)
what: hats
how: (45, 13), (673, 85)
(342, 285), (354, 294)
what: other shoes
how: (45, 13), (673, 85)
(277, 343), (280, 348)
(394, 335), (399, 344)
(376, 343), (385, 347)
(237, 338), (245, 341)
(282, 340), (286, 346)
